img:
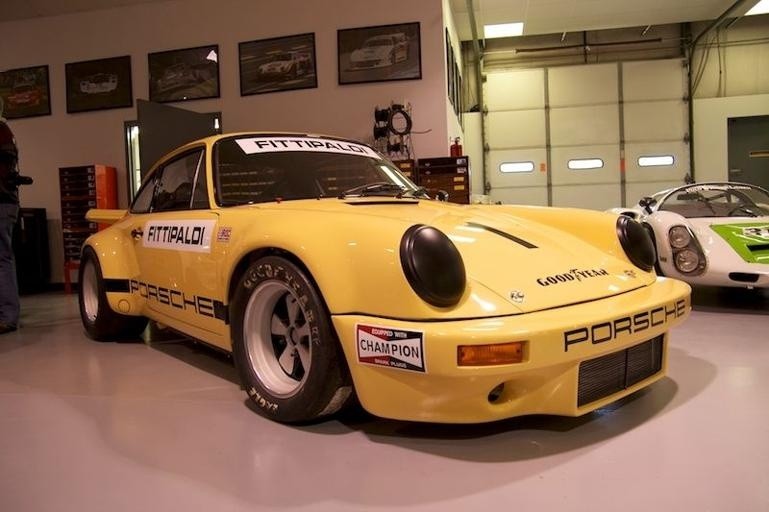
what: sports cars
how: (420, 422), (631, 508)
(157, 62), (211, 95)
(603, 181), (768, 290)
(349, 36), (410, 71)
(7, 84), (41, 112)
(80, 73), (120, 98)
(256, 50), (313, 81)
(77, 130), (695, 427)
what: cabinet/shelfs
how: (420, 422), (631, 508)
(392, 156), (470, 205)
(58, 165), (119, 295)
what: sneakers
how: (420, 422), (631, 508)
(0, 323), (17, 333)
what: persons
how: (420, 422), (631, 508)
(0, 95), (21, 334)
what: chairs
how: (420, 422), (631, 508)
(160, 168), (329, 208)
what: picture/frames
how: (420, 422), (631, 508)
(64, 54), (134, 114)
(237, 31), (319, 99)
(1, 63), (52, 121)
(147, 43), (221, 103)
(336, 21), (423, 88)
